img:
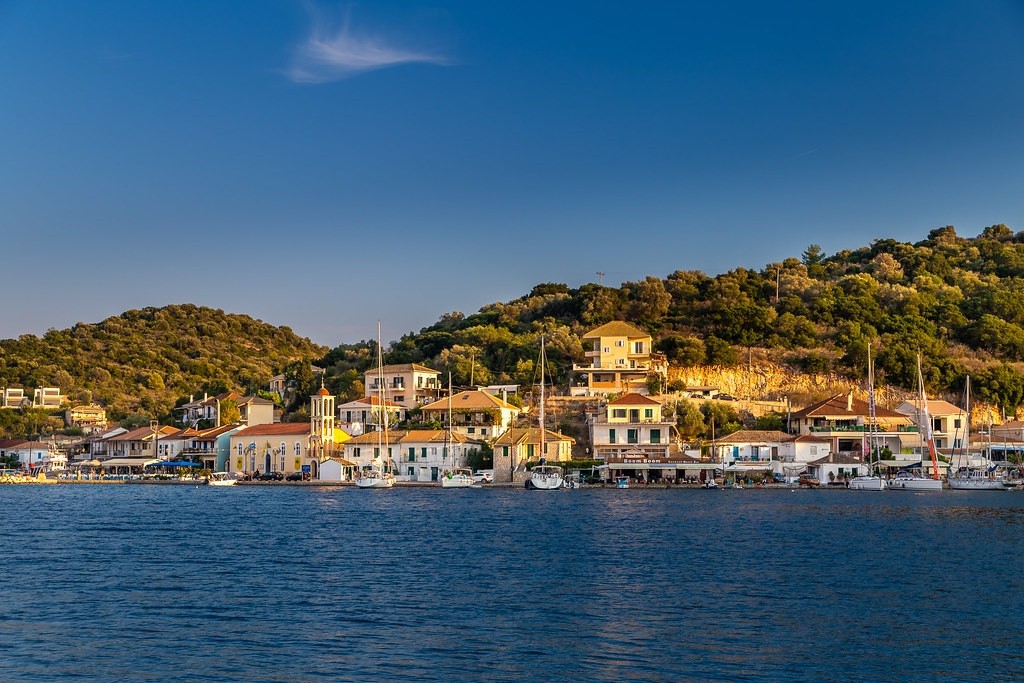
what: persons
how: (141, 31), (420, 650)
(635, 473), (774, 488)
(74, 467), (199, 483)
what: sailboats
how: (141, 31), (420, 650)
(439, 371), (477, 487)
(849, 341), (1024, 491)
(523, 334), (563, 491)
(355, 319), (394, 488)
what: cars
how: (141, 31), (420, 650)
(896, 470), (913, 478)
(285, 471), (312, 481)
(772, 473), (786, 483)
(236, 472), (249, 481)
(798, 474), (821, 486)
(257, 471), (284, 481)
(473, 472), (493, 484)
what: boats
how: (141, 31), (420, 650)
(205, 472), (238, 486)
(701, 479), (718, 488)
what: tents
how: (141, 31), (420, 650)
(72, 458), (163, 475)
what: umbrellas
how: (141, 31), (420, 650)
(149, 460), (201, 474)
